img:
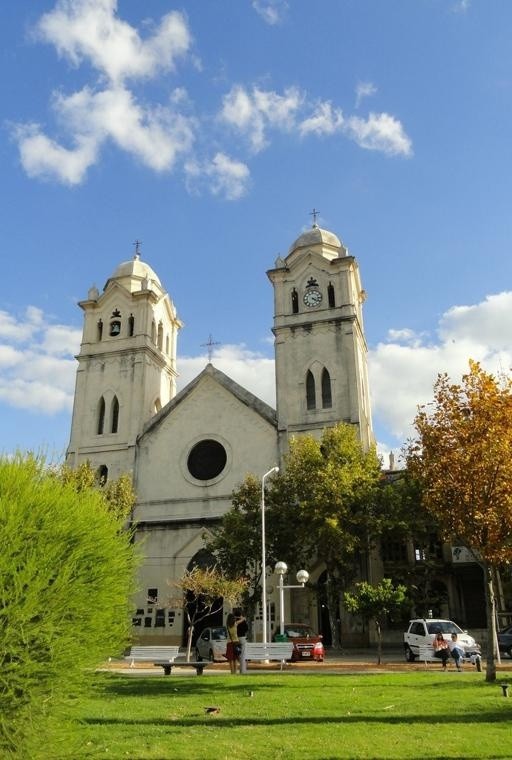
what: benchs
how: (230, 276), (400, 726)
(419, 644), (481, 668)
(154, 662), (212, 675)
(240, 642), (294, 670)
(125, 646), (180, 668)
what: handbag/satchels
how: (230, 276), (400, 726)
(234, 643), (241, 655)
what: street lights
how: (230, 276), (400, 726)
(262, 466), (279, 644)
(274, 561), (310, 636)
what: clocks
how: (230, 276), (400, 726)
(303, 290), (322, 307)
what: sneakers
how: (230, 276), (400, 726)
(443, 666), (463, 672)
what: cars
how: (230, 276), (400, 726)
(195, 626), (233, 663)
(403, 619), (483, 664)
(497, 626), (512, 658)
(271, 623), (324, 663)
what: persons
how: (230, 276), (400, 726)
(431, 632), (451, 672)
(225, 612), (247, 675)
(234, 610), (249, 675)
(447, 632), (479, 673)
